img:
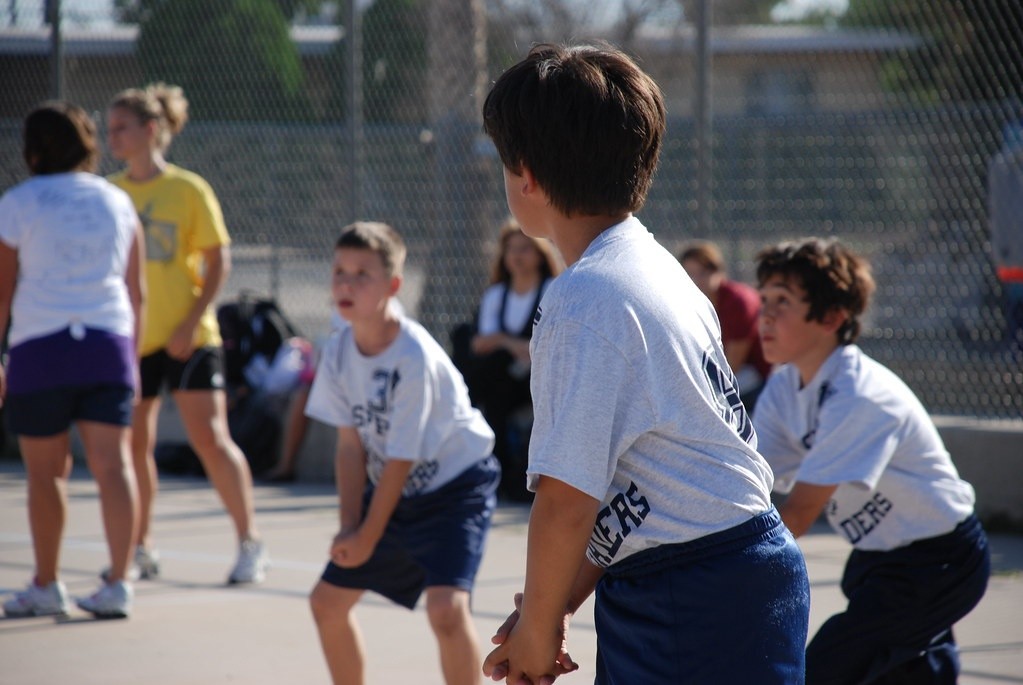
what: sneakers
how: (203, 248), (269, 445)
(77, 569), (132, 617)
(3, 578), (69, 615)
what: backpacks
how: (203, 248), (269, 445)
(217, 294), (296, 382)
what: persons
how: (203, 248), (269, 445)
(746, 238), (991, 685)
(309, 222), (502, 685)
(451, 217), (569, 506)
(93, 78), (259, 586)
(674, 240), (775, 404)
(0, 101), (147, 621)
(482, 38), (809, 685)
(226, 334), (323, 481)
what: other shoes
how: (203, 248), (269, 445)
(128, 547), (161, 579)
(230, 541), (268, 583)
(258, 465), (295, 485)
